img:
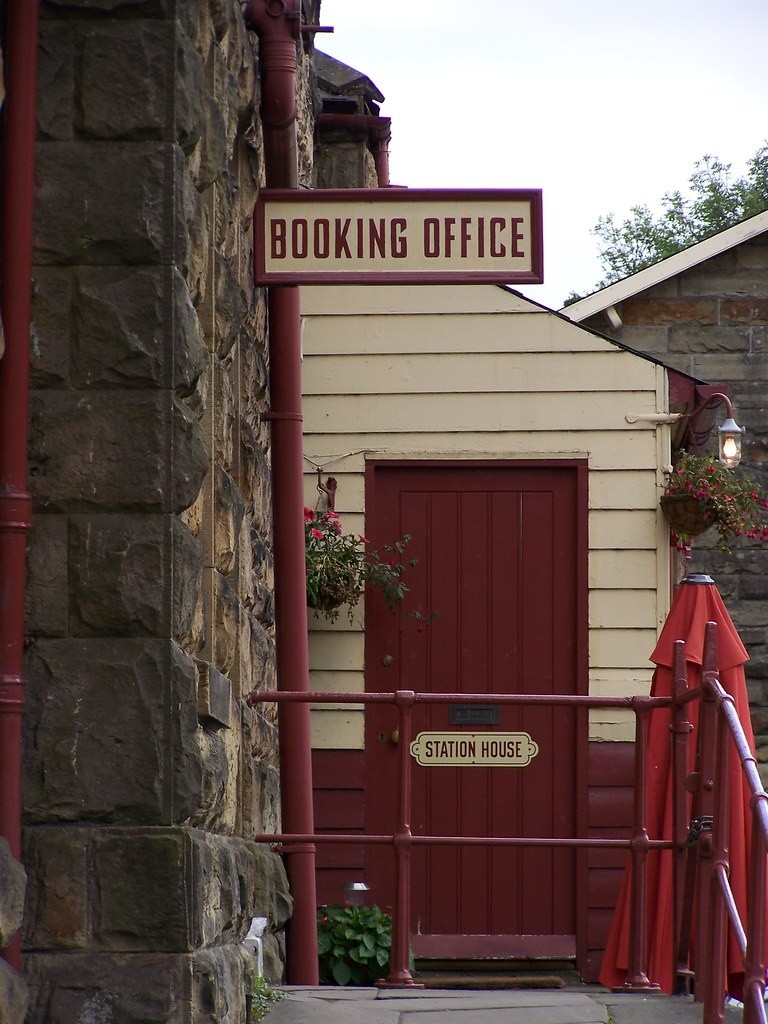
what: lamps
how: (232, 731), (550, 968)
(680, 392), (745, 469)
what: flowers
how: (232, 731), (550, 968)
(663, 452), (768, 562)
(303, 506), (425, 624)
(317, 901), (415, 985)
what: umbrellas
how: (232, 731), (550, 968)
(600, 573), (767, 1002)
